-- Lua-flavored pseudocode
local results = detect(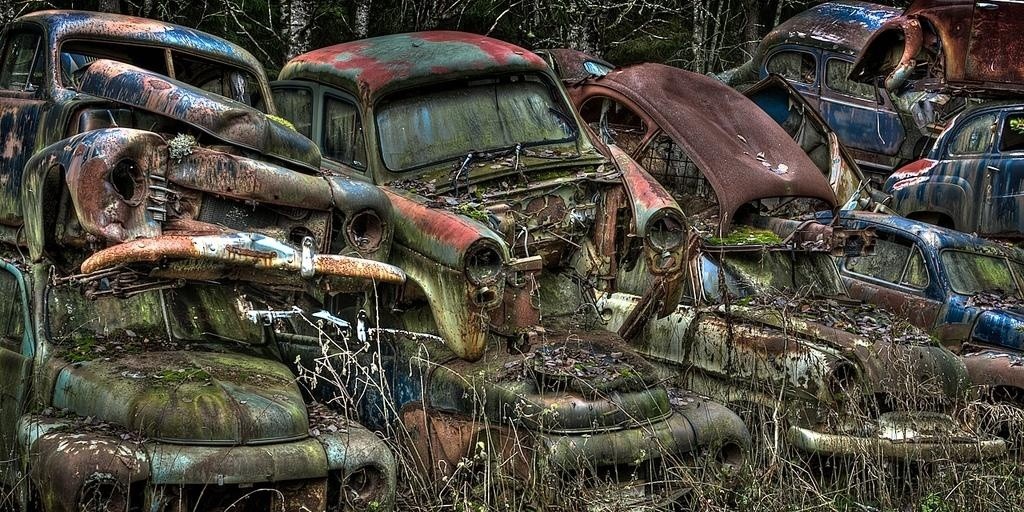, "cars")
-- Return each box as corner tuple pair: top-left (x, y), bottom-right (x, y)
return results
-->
(0, 0), (1024, 512)
